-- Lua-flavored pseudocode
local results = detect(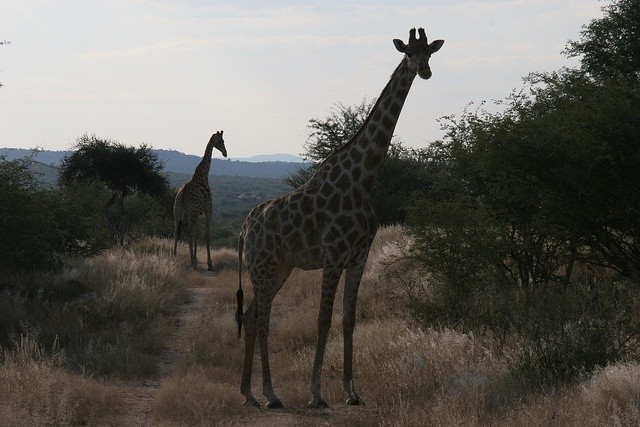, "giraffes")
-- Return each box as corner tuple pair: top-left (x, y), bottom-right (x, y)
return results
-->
(235, 27), (445, 408)
(173, 130), (227, 271)
